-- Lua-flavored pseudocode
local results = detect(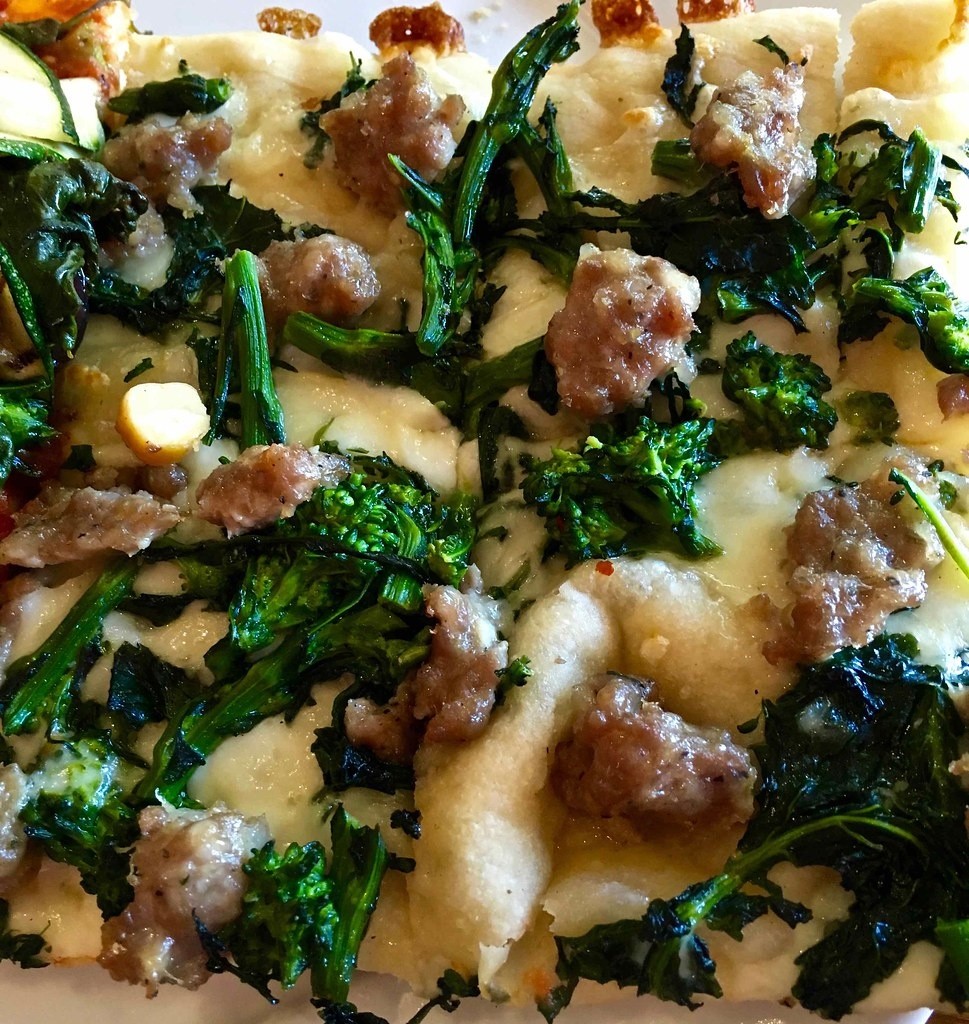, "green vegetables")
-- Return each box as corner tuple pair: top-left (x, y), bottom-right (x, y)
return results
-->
(0, 0), (969, 1024)
